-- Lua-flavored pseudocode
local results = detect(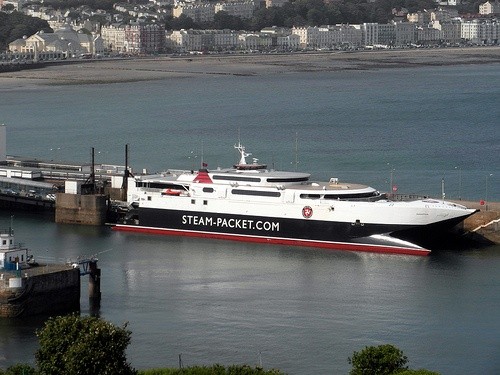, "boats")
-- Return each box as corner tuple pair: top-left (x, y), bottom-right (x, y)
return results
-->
(132, 123), (480, 256)
